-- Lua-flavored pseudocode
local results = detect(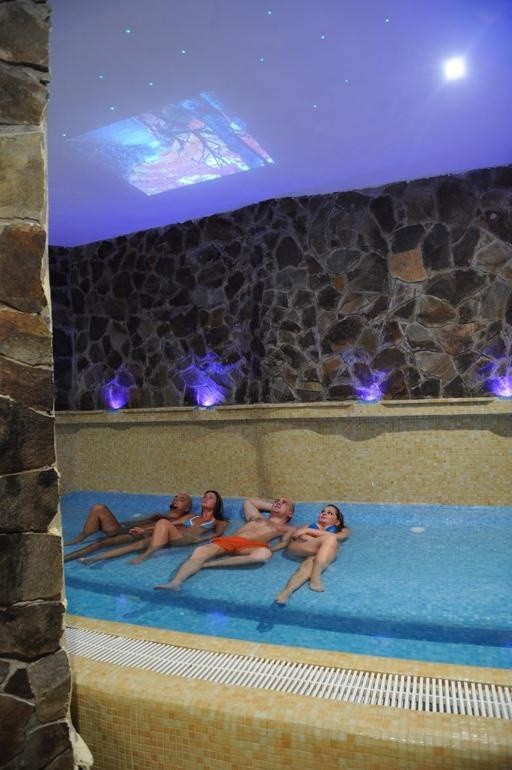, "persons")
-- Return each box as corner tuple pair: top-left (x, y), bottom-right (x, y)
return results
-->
(77, 488), (231, 568)
(152, 496), (298, 592)
(63, 492), (192, 565)
(273, 503), (350, 606)
